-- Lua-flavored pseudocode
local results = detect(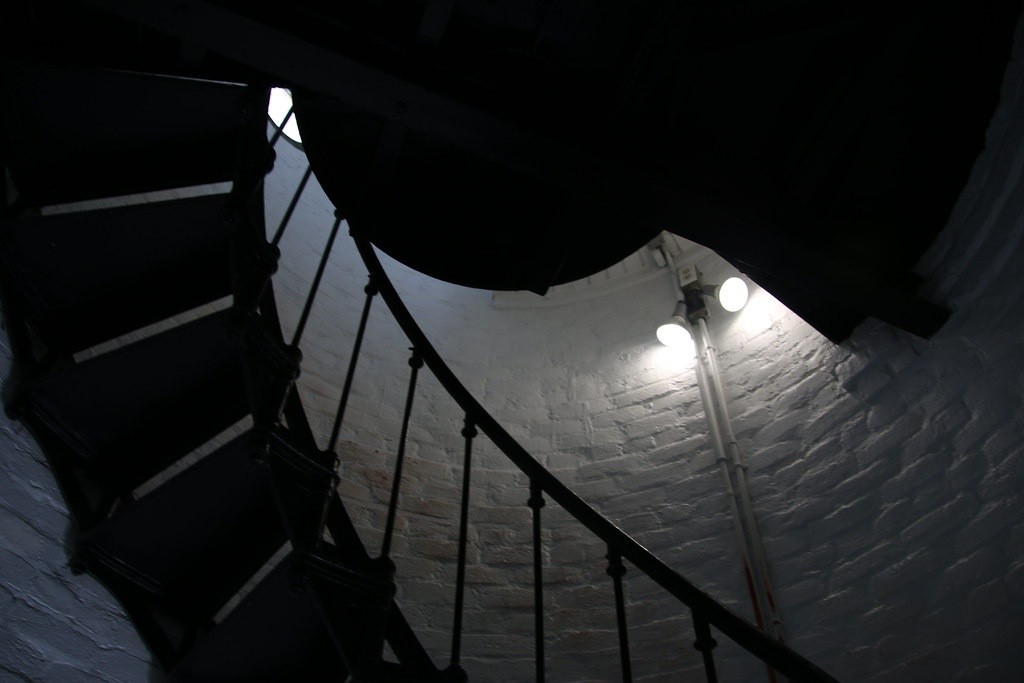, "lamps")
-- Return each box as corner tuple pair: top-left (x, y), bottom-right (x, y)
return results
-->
(701, 276), (749, 312)
(656, 300), (694, 348)
